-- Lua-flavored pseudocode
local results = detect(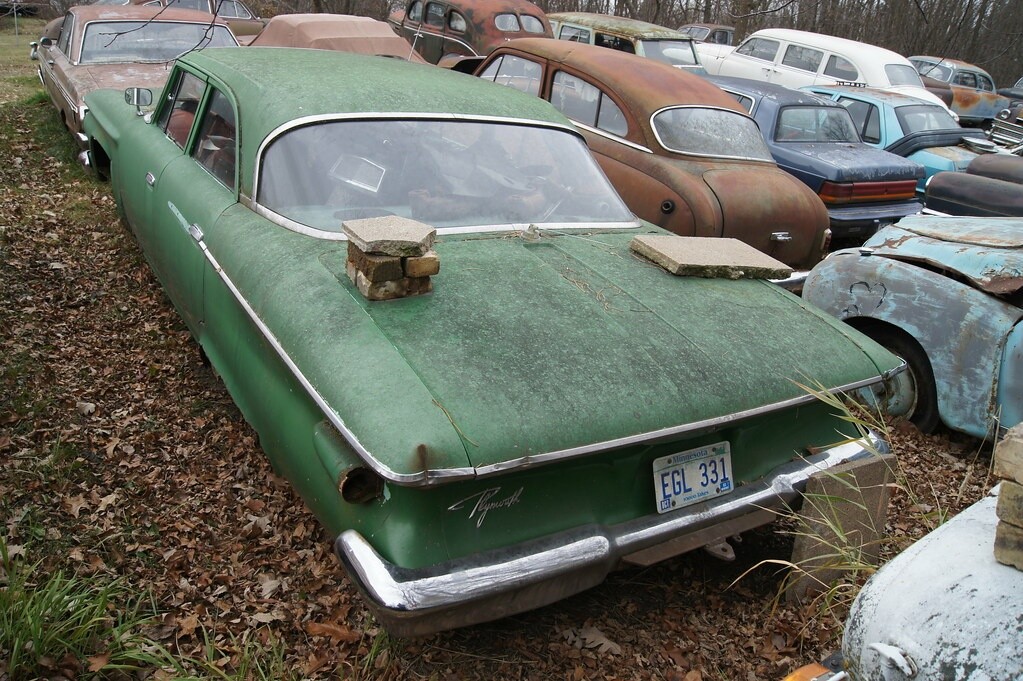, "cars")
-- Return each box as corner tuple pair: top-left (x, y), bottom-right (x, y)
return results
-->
(987, 102), (1023, 146)
(29, 5), (242, 151)
(908, 56), (1010, 125)
(662, 27), (960, 122)
(386, 0), (554, 69)
(797, 85), (1020, 206)
(236, 13), (426, 63)
(544, 12), (705, 73)
(678, 25), (751, 45)
(925, 155), (1023, 217)
(695, 74), (927, 235)
(78, 46), (911, 634)
(996, 76), (1023, 102)
(93, 0), (264, 36)
(471, 38), (831, 292)
(802, 213), (1022, 441)
